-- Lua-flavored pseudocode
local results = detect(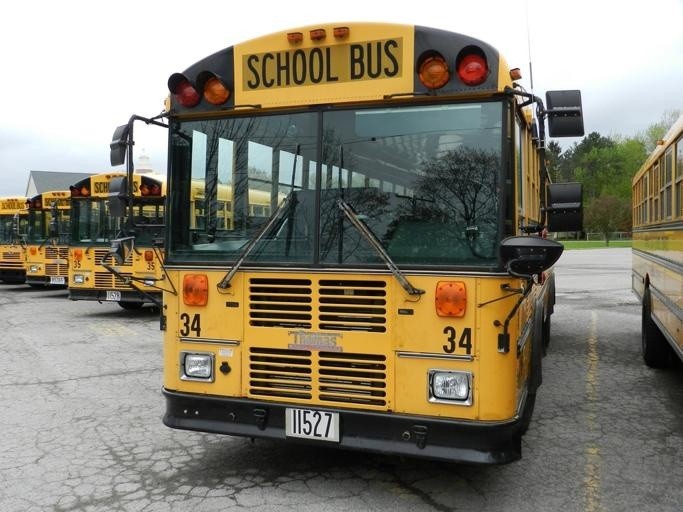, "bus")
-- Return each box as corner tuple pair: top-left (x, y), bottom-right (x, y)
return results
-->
(627, 114), (682, 372)
(0, 190), (164, 290)
(52, 173), (288, 310)
(109, 12), (595, 476)
